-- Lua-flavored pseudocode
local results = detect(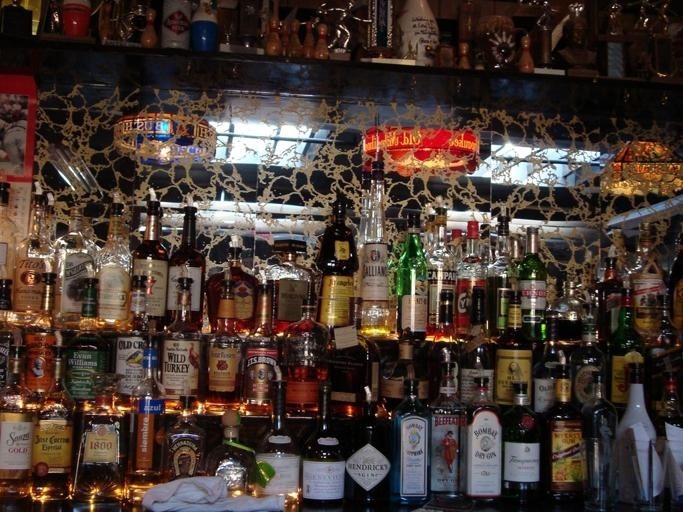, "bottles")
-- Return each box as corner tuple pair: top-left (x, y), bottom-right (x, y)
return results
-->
(0, 162), (683, 336)
(53, 2), (677, 80)
(0, 269), (427, 511)
(400, 283), (683, 511)
